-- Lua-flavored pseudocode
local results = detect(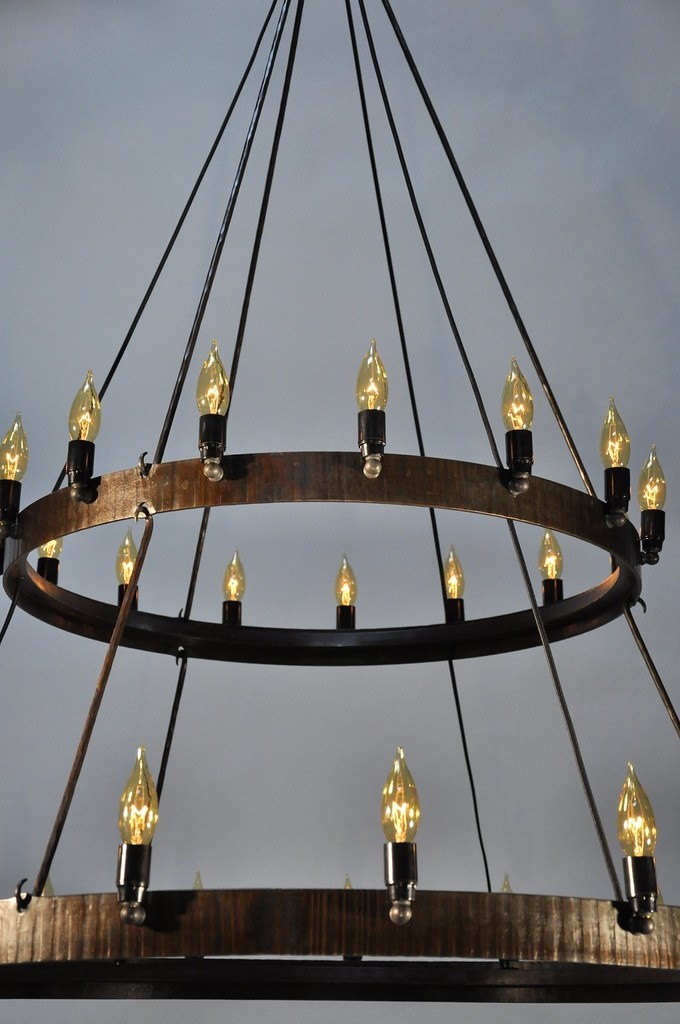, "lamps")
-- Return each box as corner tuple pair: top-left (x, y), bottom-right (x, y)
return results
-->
(0, 0), (680, 1003)
